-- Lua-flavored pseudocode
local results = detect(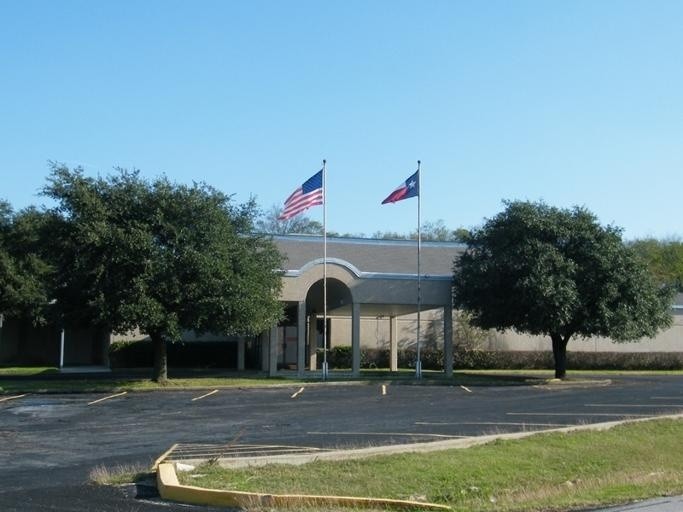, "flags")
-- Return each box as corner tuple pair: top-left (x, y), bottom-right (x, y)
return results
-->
(278, 169), (323, 222)
(381, 168), (418, 204)
